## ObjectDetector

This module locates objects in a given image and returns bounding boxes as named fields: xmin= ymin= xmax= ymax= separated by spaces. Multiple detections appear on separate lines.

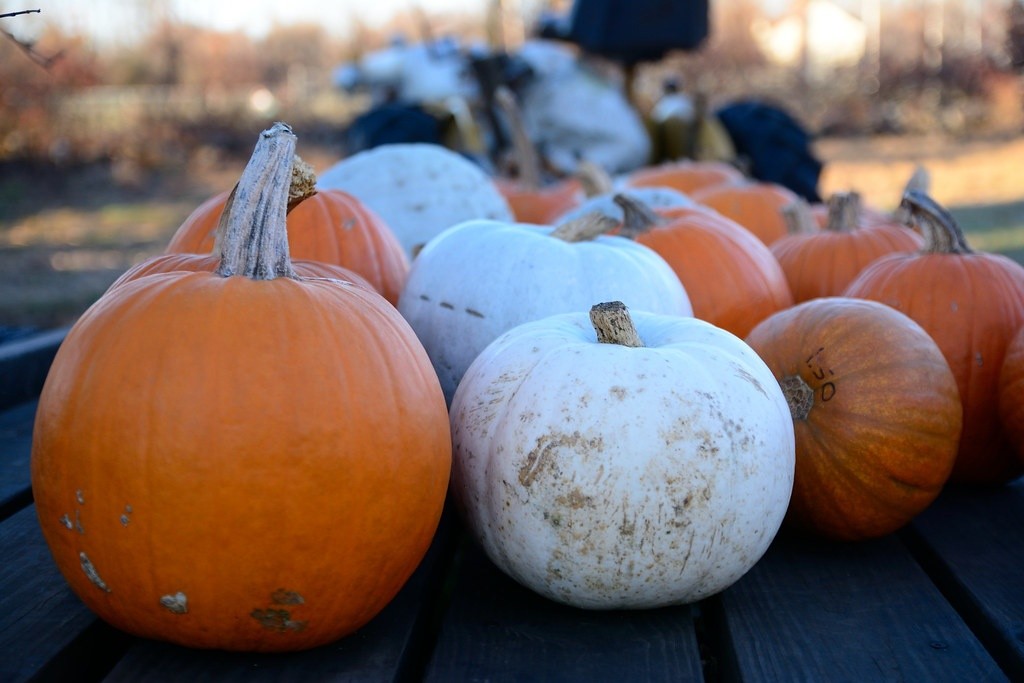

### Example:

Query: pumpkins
xmin=28 ymin=94 xmax=1024 ymax=649
